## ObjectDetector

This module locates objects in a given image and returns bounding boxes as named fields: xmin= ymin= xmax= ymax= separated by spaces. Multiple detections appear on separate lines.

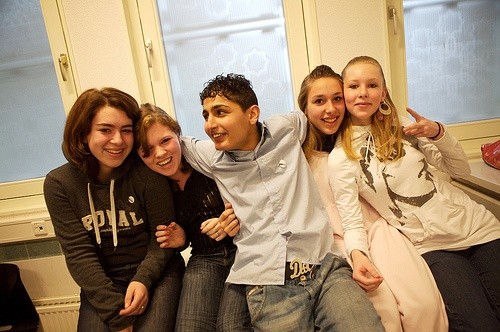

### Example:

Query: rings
xmin=142 ymin=306 xmax=145 ymax=309
xmin=216 ymin=232 xmax=220 ymax=236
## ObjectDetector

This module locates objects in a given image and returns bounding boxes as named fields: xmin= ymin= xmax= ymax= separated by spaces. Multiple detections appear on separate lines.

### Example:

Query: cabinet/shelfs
xmin=450 ymin=158 xmax=500 ymax=221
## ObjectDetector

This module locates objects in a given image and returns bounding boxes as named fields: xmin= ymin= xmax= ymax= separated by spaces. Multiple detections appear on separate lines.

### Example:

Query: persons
xmin=135 ymin=103 xmax=251 ymax=332
xmin=181 ymin=76 xmax=384 ymax=332
xmin=44 ymin=88 xmax=185 ymax=332
xmin=328 ymin=56 xmax=500 ymax=332
xmin=298 ymin=65 xmax=448 ymax=332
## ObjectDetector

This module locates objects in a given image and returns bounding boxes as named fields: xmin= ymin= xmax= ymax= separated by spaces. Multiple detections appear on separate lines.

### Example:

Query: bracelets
xmin=429 ymin=122 xmax=441 ymax=139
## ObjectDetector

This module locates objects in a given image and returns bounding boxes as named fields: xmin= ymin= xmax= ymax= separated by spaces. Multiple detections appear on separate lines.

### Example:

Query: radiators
xmin=31 ymin=294 xmax=81 ymax=332
